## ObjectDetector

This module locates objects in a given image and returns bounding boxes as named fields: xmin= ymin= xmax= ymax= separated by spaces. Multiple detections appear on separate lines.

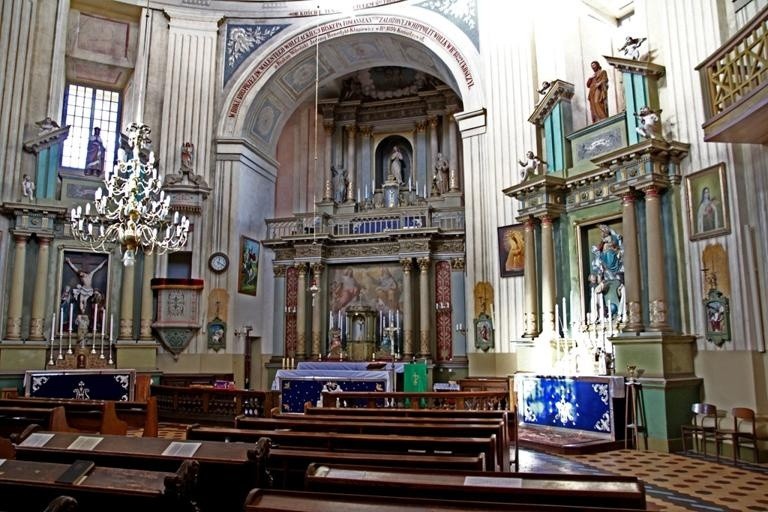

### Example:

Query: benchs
xmin=0 ymin=364 xmax=646 ymax=511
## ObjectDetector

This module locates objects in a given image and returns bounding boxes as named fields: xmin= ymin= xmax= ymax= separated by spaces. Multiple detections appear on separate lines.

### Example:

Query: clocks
xmin=207 ymin=251 xmax=230 ymax=273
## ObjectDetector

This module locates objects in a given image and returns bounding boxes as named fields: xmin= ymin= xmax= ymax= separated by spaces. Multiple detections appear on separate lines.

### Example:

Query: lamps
xmin=65 ymin=0 xmax=193 ymax=267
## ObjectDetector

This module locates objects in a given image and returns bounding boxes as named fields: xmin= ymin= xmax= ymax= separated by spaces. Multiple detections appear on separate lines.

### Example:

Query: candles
xmin=94 ymin=303 xmax=98 ymax=330
xmin=357 ymin=189 xmax=361 ymax=203
xmin=110 ymin=313 xmax=114 ymax=339
xmin=424 ymin=183 xmax=427 ymax=200
xmin=372 ymin=179 xmax=375 ymax=194
xmin=365 ymin=184 xmax=368 ymax=199
xmin=101 ymin=308 xmax=106 ymax=336
xmin=68 ymin=302 xmax=73 ymax=329
xmin=59 ymin=307 xmax=63 ymax=336
xmin=328 ymin=310 xmax=349 ymax=337
xmin=553 ymin=282 xmax=631 ymax=355
xmin=408 ymin=178 xmax=412 ymax=192
xmin=415 ymin=180 xmax=419 ymax=195
xmin=52 ymin=311 xmax=55 ymax=341
xmin=379 ymin=309 xmax=401 ymax=336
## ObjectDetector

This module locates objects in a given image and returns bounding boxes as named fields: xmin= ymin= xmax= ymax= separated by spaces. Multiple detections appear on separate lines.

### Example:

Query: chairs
xmin=680 ymin=399 xmax=720 ymax=462
xmin=714 ymin=403 xmax=760 ymax=465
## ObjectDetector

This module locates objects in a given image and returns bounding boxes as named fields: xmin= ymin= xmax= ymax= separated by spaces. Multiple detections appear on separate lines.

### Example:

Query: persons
xmin=434 ymin=152 xmax=449 ymax=196
xmin=84 ymin=127 xmax=106 ymax=177
xmin=695 ymin=186 xmax=720 ymax=232
xmin=588 ymin=224 xmax=625 ymax=319
xmin=633 ymin=104 xmax=663 ymax=140
xmin=617 ymin=36 xmax=647 ymax=61
xmin=516 ymin=149 xmax=548 ymax=182
xmin=534 ymin=80 xmax=554 ymax=108
xmin=22 ymin=173 xmax=35 ymax=200
xmin=390 ymin=146 xmax=403 ymax=185
xmin=329 ymin=263 xmax=404 ymax=354
xmin=587 ymin=61 xmax=609 ymax=123
xmin=332 ymin=161 xmax=348 ymax=204
xmin=62 ymin=257 xmax=107 ymax=334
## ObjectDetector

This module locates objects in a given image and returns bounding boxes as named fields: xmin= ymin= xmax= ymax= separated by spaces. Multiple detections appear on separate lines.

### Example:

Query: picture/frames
xmin=700 ymin=288 xmax=731 ymax=347
xmin=207 ymin=317 xmax=227 ymax=352
xmin=53 ymin=245 xmax=112 ymax=338
xmin=471 ymin=312 xmax=495 ymax=352
xmin=236 ymin=232 xmax=262 ymax=297
xmin=495 ymin=222 xmax=526 ymax=278
xmin=683 ymin=161 xmax=733 ymax=240
